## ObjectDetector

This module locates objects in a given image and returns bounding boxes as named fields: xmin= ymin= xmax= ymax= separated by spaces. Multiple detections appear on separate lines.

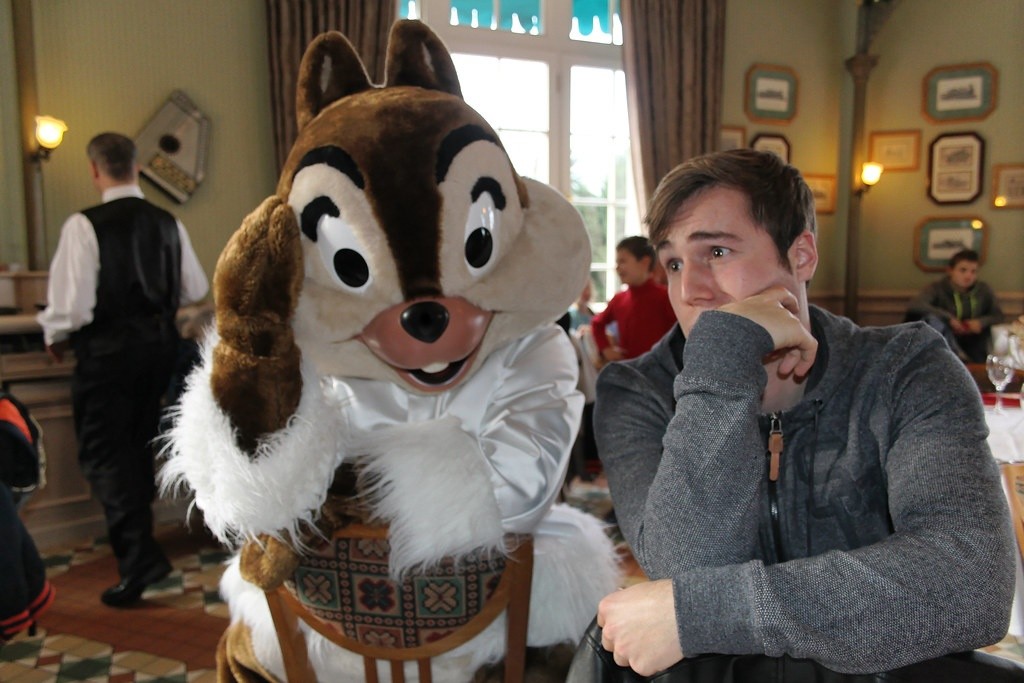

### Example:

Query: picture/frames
xmin=921 ymin=62 xmax=998 ymax=124
xmin=721 ymin=125 xmax=746 ymax=150
xmin=749 ymin=133 xmax=791 ymax=165
xmin=913 ymin=215 xmax=989 ymax=272
xmin=800 ymin=173 xmax=838 ymax=215
xmin=744 ymin=64 xmax=801 ymax=125
xmin=990 ymin=163 xmax=1024 ymax=209
xmin=926 ymin=132 xmax=986 ymax=206
xmin=868 ymin=130 xmax=921 ymax=171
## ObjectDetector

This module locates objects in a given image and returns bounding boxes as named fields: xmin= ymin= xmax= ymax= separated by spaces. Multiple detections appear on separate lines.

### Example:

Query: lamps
xmin=30 ymin=116 xmax=68 ymax=160
xmin=854 ymin=163 xmax=885 ymax=199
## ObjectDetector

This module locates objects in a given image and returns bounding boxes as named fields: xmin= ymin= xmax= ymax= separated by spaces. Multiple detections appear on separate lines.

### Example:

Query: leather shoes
xmin=102 ymin=559 xmax=172 ymax=604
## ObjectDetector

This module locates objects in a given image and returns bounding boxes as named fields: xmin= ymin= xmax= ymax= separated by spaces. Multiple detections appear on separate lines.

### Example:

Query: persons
xmin=35 ymin=132 xmax=209 ymax=607
xmin=909 ymin=250 xmax=1004 ymax=364
xmin=594 ymin=148 xmax=1016 ymax=674
xmin=554 ymin=237 xmax=678 ymax=490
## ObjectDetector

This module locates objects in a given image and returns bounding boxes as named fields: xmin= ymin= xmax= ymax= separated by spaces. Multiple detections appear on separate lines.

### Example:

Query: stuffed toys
xmin=158 ymin=20 xmax=619 ymax=683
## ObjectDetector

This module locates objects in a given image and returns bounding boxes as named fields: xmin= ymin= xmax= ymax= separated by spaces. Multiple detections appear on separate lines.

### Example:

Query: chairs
xmin=265 ymin=524 xmax=533 ymax=683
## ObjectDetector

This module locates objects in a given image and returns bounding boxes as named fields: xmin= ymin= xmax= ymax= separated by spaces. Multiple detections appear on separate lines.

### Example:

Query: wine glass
xmin=985 ymin=354 xmax=1015 ymax=415
